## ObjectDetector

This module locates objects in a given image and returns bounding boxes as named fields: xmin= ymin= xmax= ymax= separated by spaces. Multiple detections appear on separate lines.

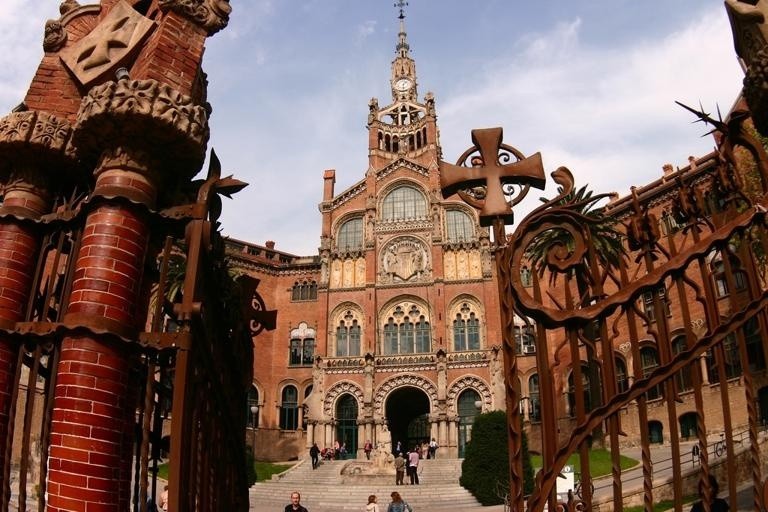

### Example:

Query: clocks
xmin=393 ymin=77 xmax=414 ymax=92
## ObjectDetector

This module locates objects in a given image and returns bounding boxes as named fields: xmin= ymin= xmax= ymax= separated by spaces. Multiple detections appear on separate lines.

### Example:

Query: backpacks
xmin=311 ymin=448 xmax=316 ymax=456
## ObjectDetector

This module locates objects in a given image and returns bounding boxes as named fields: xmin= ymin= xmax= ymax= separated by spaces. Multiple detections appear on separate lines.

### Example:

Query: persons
xmin=404 ymin=450 xmax=411 ymax=485
xmin=688 ymin=472 xmax=730 ymax=511
xmin=396 ymin=451 xmax=405 ymax=484
xmin=408 ymin=445 xmax=419 ymax=485
xmin=386 ymin=491 xmax=412 ymax=512
xmin=365 ymin=494 xmax=380 ymax=512
xmin=284 ymin=492 xmax=308 ymax=511
xmin=310 ymin=441 xmax=321 ymax=469
xmin=320 ymin=438 xmax=348 ymax=460
xmin=364 ymin=438 xmax=373 ymax=461
xmin=394 ymin=436 xmax=438 ymax=459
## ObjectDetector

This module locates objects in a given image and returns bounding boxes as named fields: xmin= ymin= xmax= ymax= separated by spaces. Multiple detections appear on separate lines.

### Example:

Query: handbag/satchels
xmin=404 ymin=505 xmax=409 ymax=512
xmin=435 ymin=444 xmax=439 ymax=449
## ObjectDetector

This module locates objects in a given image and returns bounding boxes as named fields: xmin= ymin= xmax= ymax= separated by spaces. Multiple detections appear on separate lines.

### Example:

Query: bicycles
xmin=574 ymin=472 xmax=595 ymax=500
xmin=715 ymin=431 xmax=740 ymax=458
